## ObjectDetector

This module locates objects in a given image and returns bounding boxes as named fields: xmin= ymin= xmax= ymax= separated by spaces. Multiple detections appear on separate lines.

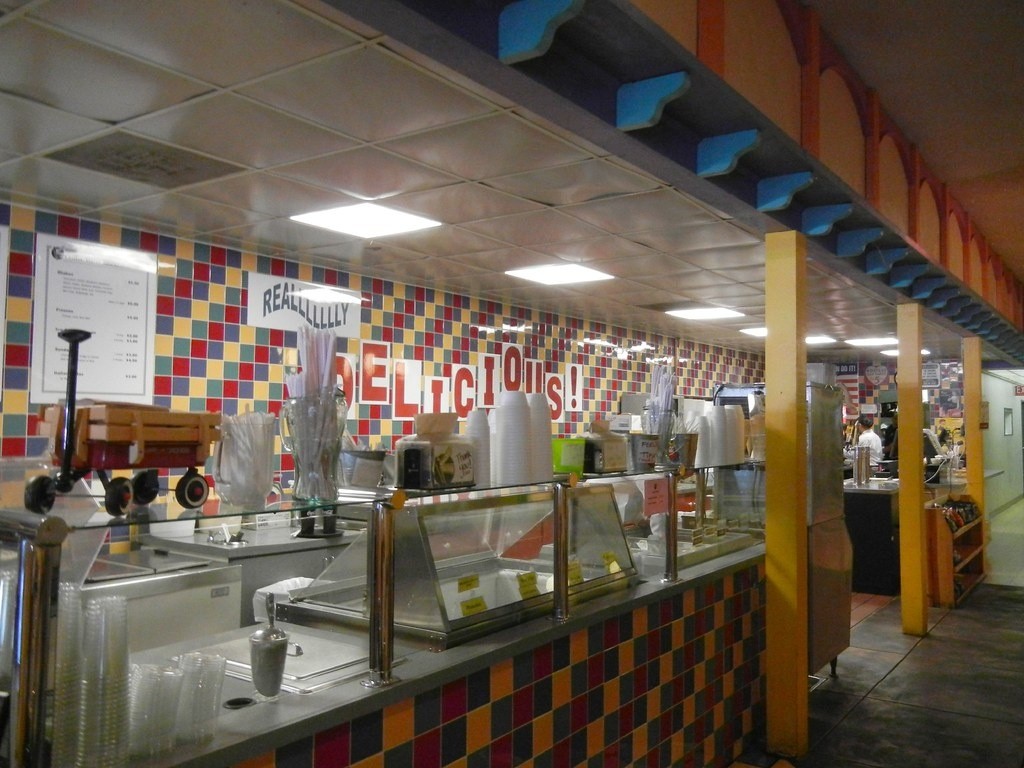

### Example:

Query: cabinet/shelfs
xmin=923 ymin=493 xmax=989 ymax=609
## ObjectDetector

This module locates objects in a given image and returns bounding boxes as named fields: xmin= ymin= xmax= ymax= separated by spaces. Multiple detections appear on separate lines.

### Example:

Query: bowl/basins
xmin=462 ymin=391 xmax=556 ymax=490
xmin=685 ymin=405 xmax=745 ymax=467
xmin=930 ymin=458 xmax=940 ymax=464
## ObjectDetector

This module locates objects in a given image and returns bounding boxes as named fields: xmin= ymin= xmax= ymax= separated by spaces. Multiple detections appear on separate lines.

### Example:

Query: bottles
xmin=852 ymin=445 xmax=870 ymax=485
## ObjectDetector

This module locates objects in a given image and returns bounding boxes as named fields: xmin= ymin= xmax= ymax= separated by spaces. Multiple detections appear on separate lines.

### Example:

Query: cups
xmin=279 ymin=388 xmax=349 ymax=503
xmin=216 ymin=412 xmax=277 ymax=516
xmin=250 ymin=626 xmax=289 ymax=703
xmin=672 ymin=432 xmax=699 ymax=470
xmin=548 ymin=435 xmax=586 ymax=478
xmin=641 ymin=406 xmax=675 ymax=470
xmin=631 ymin=432 xmax=659 ymax=471
xmin=0 ymin=568 xmax=229 ymax=768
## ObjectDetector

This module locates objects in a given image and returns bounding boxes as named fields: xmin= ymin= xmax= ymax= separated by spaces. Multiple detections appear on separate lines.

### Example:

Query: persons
xmin=843 ymin=414 xmax=883 ymax=478
xmin=881 ymin=413 xmax=898 ymax=460
